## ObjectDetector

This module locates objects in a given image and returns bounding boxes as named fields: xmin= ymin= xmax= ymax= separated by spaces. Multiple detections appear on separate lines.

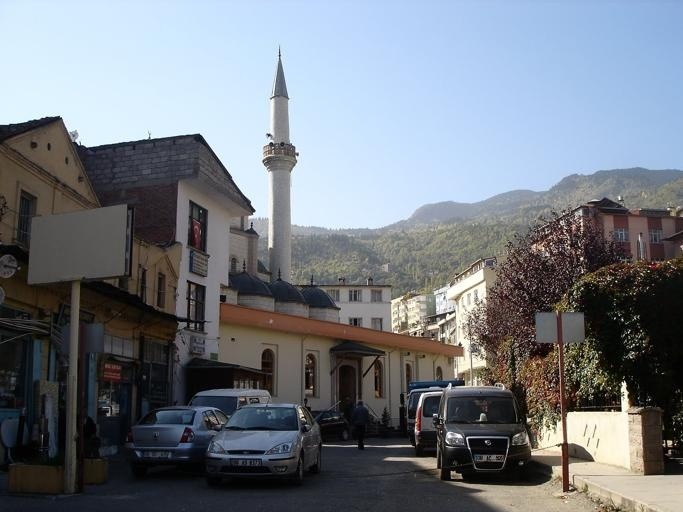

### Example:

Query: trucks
xmin=400 ymin=380 xmax=465 ymax=446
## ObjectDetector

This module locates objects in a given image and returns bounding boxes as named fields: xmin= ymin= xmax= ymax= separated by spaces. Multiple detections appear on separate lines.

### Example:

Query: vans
xmin=415 ymin=392 xmax=444 ymax=457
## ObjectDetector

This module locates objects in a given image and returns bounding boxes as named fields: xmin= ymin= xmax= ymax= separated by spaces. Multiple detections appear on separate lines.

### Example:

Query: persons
xmin=350 ymin=400 xmax=370 ymax=450
xmin=82 ymin=415 xmax=100 ymax=459
xmin=343 ymin=391 xmax=354 ymax=440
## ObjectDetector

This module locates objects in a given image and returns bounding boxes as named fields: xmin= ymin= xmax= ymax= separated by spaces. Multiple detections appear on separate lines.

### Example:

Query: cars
xmin=433 ymin=382 xmax=531 ymax=481
xmin=124 ymin=388 xmax=352 ymax=487
